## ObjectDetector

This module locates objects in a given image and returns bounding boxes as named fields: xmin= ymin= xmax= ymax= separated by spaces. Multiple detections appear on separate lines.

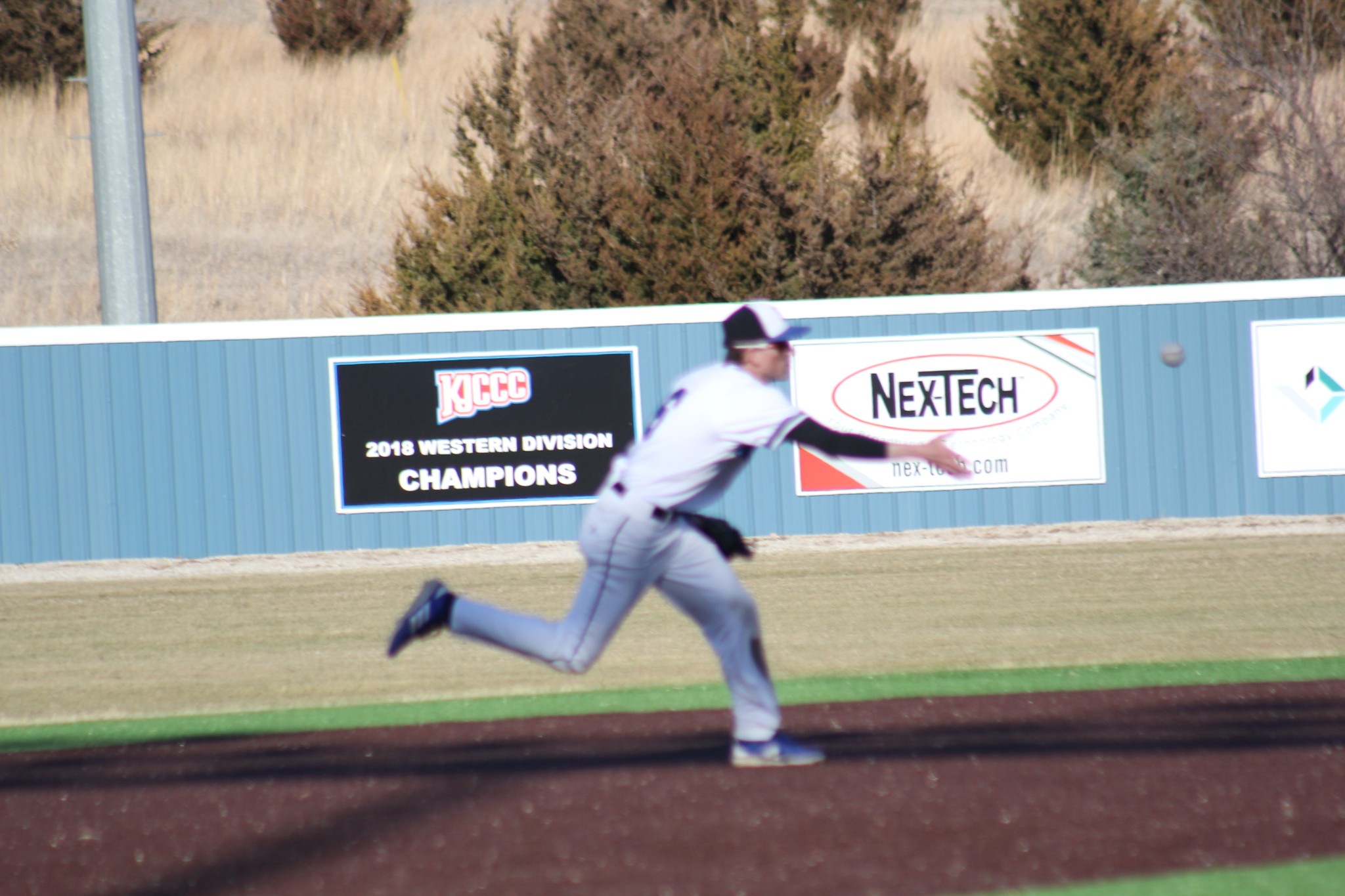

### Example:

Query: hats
xmin=722 ymin=304 xmax=811 ymax=346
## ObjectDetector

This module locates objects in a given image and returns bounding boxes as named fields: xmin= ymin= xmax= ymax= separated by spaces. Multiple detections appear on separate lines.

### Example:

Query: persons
xmin=389 ymin=303 xmax=972 ymax=770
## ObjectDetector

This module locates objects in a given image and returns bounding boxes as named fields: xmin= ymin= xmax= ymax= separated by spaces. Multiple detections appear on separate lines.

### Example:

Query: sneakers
xmin=386 ymin=579 xmax=454 ymax=658
xmin=729 ymin=731 xmax=827 ymax=766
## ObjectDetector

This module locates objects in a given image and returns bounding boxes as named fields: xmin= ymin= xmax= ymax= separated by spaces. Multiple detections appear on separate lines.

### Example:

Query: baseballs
xmin=1160 ymin=342 xmax=1186 ymax=368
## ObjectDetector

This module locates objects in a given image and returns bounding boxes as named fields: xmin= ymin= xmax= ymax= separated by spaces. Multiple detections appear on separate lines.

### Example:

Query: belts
xmin=610 ymin=482 xmax=668 ymax=524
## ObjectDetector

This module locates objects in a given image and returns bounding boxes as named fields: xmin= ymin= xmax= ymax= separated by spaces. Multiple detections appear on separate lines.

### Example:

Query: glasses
xmin=730 ymin=339 xmax=795 ymax=355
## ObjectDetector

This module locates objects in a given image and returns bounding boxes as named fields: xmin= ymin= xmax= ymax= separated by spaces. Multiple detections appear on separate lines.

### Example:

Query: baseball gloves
xmin=693 ymin=513 xmax=754 ymax=560
